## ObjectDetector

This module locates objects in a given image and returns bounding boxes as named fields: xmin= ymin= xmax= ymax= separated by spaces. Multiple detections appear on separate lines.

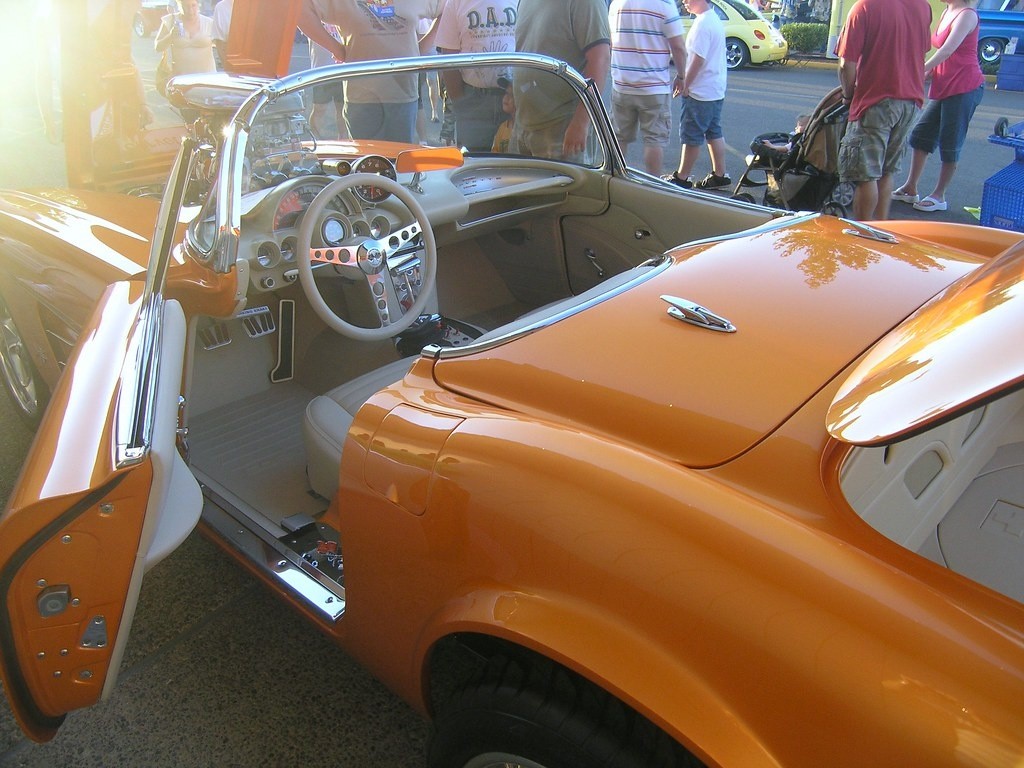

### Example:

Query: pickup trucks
xmin=973 ymin=10 xmax=1023 ymax=77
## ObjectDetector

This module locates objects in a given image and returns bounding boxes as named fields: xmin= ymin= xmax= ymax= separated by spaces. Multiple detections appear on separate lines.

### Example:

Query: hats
xmin=496 ymin=76 xmax=513 ymax=88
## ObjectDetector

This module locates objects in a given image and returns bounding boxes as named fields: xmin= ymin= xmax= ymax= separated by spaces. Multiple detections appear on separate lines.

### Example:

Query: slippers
xmin=913 ymin=195 xmax=948 ymax=213
xmin=892 ymin=187 xmax=920 ymax=204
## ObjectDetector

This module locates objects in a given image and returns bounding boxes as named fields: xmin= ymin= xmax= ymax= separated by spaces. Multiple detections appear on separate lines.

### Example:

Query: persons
xmin=891 ymin=0 xmax=986 ymax=213
xmin=435 ymin=0 xmax=519 ymax=151
xmin=762 ymin=113 xmax=811 ymax=155
xmin=295 ymin=0 xmax=446 ymax=146
xmin=608 ymin=0 xmax=687 ymax=178
xmin=167 ymin=0 xmax=178 ymax=13
xmin=503 ymin=0 xmax=611 ymax=167
xmin=154 ymin=0 xmax=217 ymax=125
xmin=307 ymin=22 xmax=347 ymax=140
xmin=833 ymin=0 xmax=933 ymax=220
xmin=209 ymin=0 xmax=234 ymax=71
xmin=37 ymin=0 xmax=153 ymax=193
xmin=660 ymin=0 xmax=732 ymax=189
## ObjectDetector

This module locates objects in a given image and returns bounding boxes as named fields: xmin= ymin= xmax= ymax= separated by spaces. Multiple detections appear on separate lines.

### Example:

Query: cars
xmin=674 ymin=0 xmax=789 ymax=69
xmin=1 ymin=51 xmax=1024 ymax=767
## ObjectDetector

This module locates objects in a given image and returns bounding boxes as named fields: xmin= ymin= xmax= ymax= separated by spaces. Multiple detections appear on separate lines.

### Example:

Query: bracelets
xmin=677 ymin=75 xmax=684 ymax=80
xmin=841 ymin=93 xmax=853 ymax=100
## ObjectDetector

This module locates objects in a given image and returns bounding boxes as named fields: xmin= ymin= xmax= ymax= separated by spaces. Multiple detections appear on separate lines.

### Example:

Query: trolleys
xmin=980 ymin=117 xmax=1023 ymax=231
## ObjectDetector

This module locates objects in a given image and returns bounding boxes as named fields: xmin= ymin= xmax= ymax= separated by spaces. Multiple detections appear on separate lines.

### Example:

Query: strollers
xmin=726 ymin=85 xmax=855 ymax=224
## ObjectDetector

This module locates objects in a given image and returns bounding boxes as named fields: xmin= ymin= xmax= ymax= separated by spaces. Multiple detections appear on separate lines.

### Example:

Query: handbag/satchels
xmin=155 ymin=49 xmax=174 ymax=97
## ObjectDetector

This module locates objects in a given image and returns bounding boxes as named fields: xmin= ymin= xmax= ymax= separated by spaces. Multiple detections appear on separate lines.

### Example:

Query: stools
xmin=300 ymin=352 xmax=425 ymax=503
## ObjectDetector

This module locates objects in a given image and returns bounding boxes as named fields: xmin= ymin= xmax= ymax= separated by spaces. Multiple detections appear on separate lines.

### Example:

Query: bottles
xmin=1004 ymin=43 xmax=1010 ymax=53
xmin=175 ymin=18 xmax=184 ymax=38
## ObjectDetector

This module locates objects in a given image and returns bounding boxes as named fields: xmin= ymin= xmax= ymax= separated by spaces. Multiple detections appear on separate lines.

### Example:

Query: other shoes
xmin=430 ymin=118 xmax=440 ymax=122
xmin=418 ymin=141 xmax=428 ymax=146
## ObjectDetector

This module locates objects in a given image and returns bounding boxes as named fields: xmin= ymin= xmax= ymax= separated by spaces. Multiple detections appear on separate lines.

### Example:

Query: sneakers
xmin=659 ymin=172 xmax=692 ymax=189
xmin=694 ymin=171 xmax=732 ymax=190
